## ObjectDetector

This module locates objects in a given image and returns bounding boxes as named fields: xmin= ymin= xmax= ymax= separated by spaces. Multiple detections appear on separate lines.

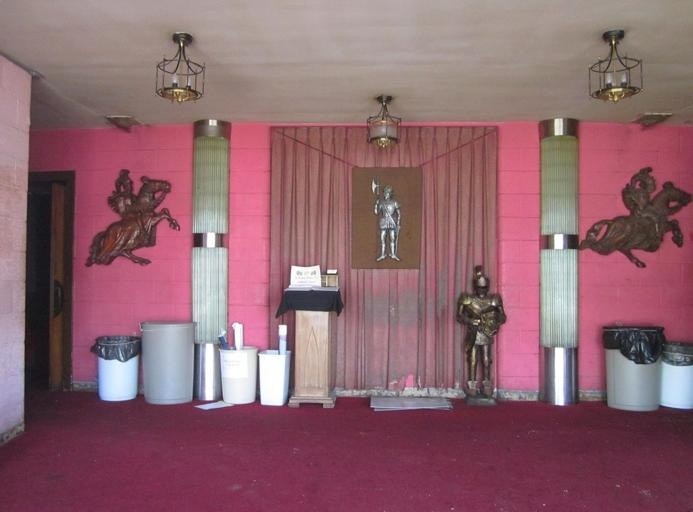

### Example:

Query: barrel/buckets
xmin=139 ymin=320 xmax=198 ymax=407
xmin=216 ymin=346 xmax=260 ymax=406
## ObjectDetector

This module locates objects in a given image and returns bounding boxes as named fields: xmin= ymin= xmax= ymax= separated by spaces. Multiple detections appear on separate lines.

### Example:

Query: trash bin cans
xmin=90 ymin=321 xmax=292 ymax=406
xmin=602 ymin=326 xmax=693 ymax=411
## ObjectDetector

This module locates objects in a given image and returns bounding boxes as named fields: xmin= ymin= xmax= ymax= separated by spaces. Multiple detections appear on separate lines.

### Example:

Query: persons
xmin=106 ymin=168 xmax=152 ymax=241
xmin=457 ymin=265 xmax=507 ymax=397
xmin=621 ymin=166 xmax=666 ymax=238
xmin=373 ymin=184 xmax=402 ymax=262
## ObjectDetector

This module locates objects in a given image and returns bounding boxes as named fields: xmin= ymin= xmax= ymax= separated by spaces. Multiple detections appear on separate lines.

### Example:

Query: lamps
xmin=364 ymin=94 xmax=401 ymax=151
xmin=153 ymin=32 xmax=208 ymax=104
xmin=537 ymin=112 xmax=579 ymax=411
xmin=190 ymin=116 xmax=232 ymax=405
xmin=588 ymin=28 xmax=645 ymax=103
